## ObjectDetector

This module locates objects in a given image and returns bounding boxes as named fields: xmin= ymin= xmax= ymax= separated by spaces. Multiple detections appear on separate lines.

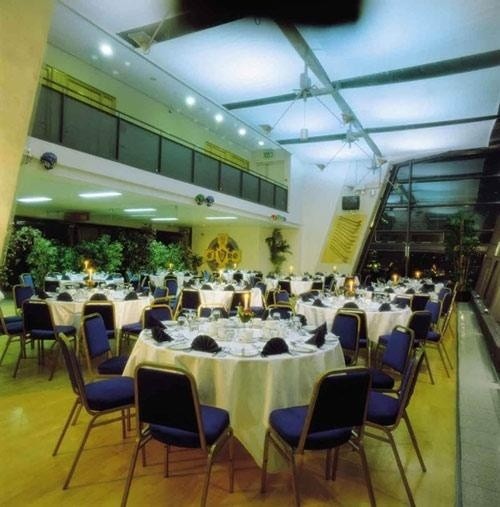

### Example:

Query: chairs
xmin=261 ymin=365 xmax=377 ymax=507
xmin=120 ymin=361 xmax=234 ymax=507
xmin=332 ymin=348 xmax=427 ymax=507
xmin=52 ymin=332 xmax=147 ymax=490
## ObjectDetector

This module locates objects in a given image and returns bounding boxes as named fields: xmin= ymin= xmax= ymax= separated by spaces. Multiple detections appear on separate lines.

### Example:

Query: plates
xmin=229 ymin=346 xmax=259 ymax=357
xmin=292 ymin=344 xmax=317 ymax=353
xmin=324 ymin=335 xmax=338 ymax=342
xmin=303 ymin=326 xmax=318 ymax=332
xmin=166 ymin=343 xmax=191 ymax=350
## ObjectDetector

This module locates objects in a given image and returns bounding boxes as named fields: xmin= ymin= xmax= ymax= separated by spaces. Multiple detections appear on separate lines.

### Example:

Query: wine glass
xmin=266 ymin=307 xmax=273 ymax=321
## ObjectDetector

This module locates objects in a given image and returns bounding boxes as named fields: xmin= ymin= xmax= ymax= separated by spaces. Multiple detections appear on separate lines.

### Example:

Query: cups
xmin=177 ymin=316 xmax=185 ymax=330
xmin=212 ymin=311 xmax=220 ymax=322
xmin=273 ymin=312 xmax=281 ymax=322
